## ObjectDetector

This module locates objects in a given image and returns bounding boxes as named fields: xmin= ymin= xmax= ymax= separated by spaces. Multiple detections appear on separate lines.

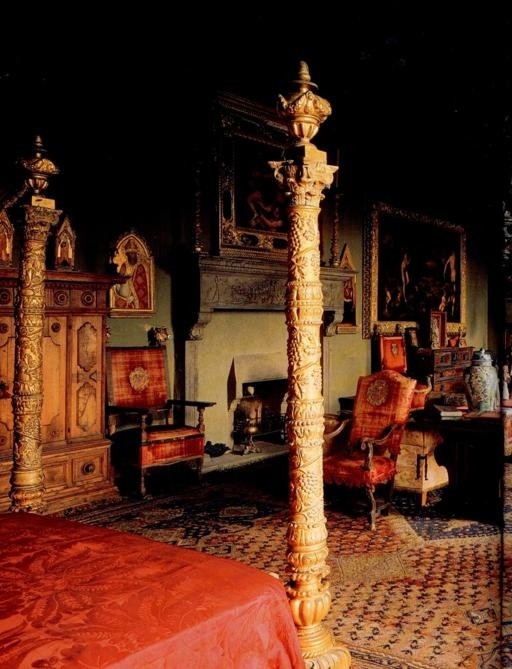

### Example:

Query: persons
xmin=113 ymin=238 xmax=148 ymax=309
xmin=444 ymin=250 xmax=456 ymax=287
xmin=400 ymin=252 xmax=411 ymax=303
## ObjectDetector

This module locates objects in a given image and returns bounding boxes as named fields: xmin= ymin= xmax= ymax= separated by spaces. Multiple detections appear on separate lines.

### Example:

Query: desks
xmin=337 ymin=390 xmax=505 ymax=531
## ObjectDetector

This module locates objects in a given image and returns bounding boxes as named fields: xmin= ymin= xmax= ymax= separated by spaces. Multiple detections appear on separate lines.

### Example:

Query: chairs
xmin=321 ymin=369 xmax=417 ymax=532
xmin=104 ymin=344 xmax=217 ymax=502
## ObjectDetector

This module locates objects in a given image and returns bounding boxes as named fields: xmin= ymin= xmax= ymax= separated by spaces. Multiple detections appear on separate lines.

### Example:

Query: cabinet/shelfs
xmin=412 ymin=346 xmax=476 ymax=393
xmin=0 ymin=267 xmax=128 ymax=516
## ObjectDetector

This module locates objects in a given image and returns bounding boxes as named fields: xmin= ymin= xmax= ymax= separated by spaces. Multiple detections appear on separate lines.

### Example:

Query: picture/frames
xmin=335 ymin=196 xmax=468 ymax=340
xmin=213 ymin=114 xmax=289 ymax=262
xmin=109 ymin=230 xmax=156 ymax=319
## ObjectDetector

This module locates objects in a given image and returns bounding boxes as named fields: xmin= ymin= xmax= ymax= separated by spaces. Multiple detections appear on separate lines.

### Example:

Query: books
xmin=432 ymin=404 xmax=463 ymax=417
